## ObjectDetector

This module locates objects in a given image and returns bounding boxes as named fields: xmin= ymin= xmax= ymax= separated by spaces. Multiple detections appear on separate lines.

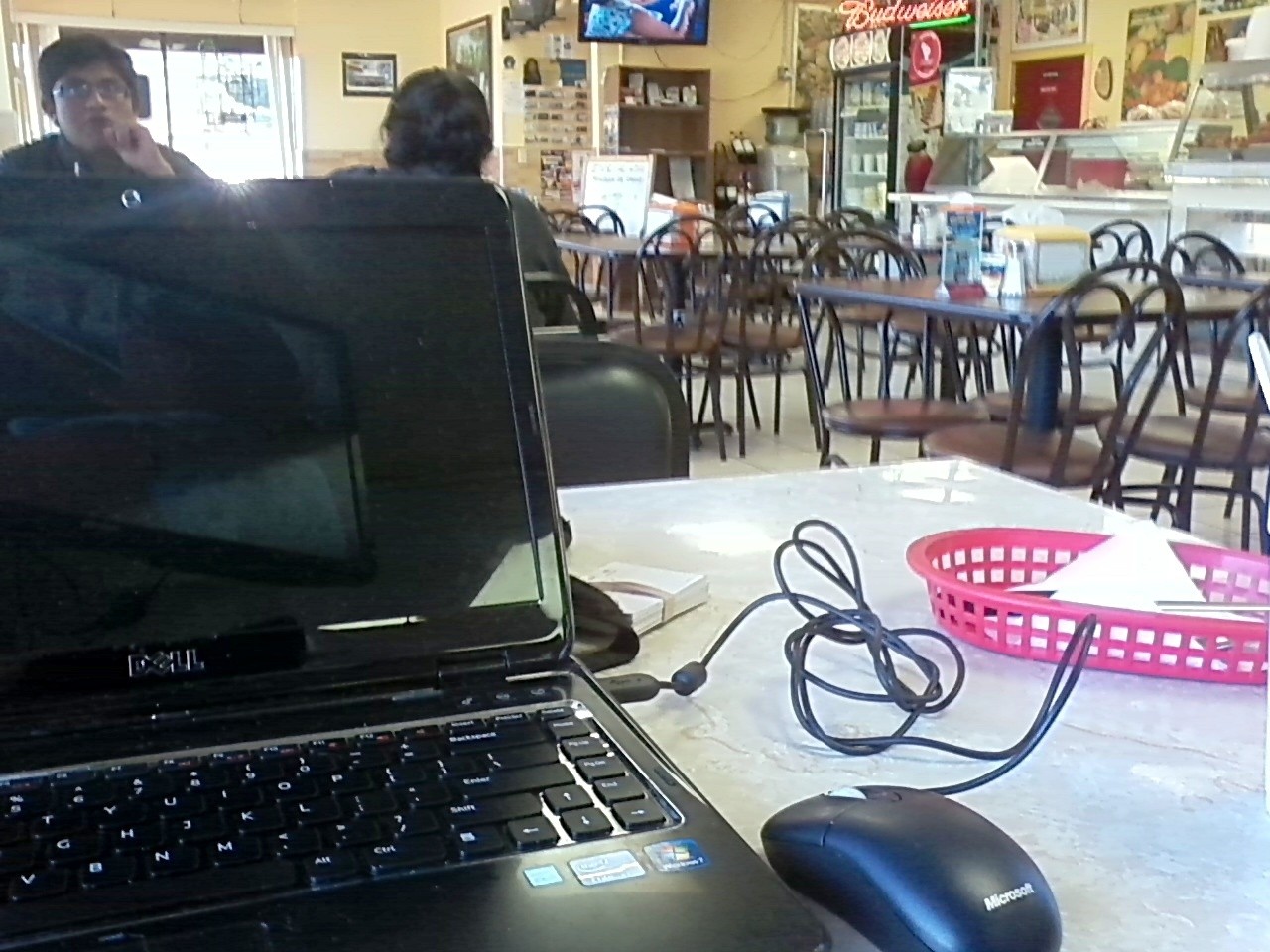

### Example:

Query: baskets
xmin=905 ymin=527 xmax=1269 ymax=687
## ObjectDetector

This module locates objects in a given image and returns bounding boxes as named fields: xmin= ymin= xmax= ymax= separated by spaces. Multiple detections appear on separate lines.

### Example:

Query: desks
xmin=553 ymin=454 xmax=1270 ymax=952
xmin=795 ymin=274 xmax=1268 ymax=429
xmin=558 ymin=231 xmax=810 ymax=440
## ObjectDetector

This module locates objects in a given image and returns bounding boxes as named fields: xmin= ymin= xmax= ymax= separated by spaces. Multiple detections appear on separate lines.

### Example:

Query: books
xmin=580 ymin=560 xmax=710 ymax=636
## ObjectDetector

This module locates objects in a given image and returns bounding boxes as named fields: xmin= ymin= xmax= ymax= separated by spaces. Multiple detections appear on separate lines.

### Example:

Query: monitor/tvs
xmin=577 ymin=0 xmax=710 ymax=45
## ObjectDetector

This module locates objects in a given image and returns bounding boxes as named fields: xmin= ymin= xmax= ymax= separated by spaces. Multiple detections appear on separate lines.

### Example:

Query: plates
xmin=834 ymin=29 xmax=887 ymax=69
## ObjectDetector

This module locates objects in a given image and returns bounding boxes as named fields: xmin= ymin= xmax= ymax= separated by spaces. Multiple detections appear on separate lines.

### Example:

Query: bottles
xmin=912 ymin=204 xmax=939 ymax=247
xmin=903 ymin=139 xmax=934 ymax=231
xmin=764 ymin=114 xmax=800 ymax=145
xmin=716 ymin=129 xmax=757 ymax=210
xmin=1001 ymin=239 xmax=1027 ymax=298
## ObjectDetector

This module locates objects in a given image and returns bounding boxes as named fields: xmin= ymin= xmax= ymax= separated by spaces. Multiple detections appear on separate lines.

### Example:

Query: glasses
xmin=52 ymin=79 xmax=131 ymax=100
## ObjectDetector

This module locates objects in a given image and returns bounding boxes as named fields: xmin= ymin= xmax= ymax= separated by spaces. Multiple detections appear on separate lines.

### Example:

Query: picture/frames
xmin=446 ymin=16 xmax=493 ymax=136
xmin=342 ymin=52 xmax=396 ymax=98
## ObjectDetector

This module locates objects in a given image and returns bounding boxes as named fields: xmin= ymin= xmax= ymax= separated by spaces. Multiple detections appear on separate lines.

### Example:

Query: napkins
xmin=990 ymin=517 xmax=1269 ymax=674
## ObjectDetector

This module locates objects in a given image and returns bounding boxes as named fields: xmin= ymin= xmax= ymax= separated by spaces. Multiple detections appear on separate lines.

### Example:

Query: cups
xmin=981 ymin=259 xmax=1003 ymax=297
xmin=1225 ymin=38 xmax=1245 ymax=62
xmin=843 ymin=80 xmax=890 ymax=216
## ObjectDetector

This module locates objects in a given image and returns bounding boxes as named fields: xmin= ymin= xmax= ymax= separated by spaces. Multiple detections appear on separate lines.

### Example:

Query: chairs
xmin=521 ymin=203 xmax=1270 ymax=562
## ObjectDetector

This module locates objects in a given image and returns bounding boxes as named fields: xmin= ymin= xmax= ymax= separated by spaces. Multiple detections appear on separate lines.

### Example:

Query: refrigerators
xmin=832 ymin=25 xmax=997 ymax=232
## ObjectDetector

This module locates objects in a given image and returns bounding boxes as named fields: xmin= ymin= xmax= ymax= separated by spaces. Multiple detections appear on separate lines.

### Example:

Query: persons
xmin=586 ymin=0 xmax=698 ymax=43
xmin=324 ymin=66 xmax=579 ymax=329
xmin=0 ymin=35 xmax=228 ymax=188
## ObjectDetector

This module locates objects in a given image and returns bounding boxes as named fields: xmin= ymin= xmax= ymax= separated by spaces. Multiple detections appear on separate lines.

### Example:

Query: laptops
xmin=1 ymin=168 xmax=834 ymax=952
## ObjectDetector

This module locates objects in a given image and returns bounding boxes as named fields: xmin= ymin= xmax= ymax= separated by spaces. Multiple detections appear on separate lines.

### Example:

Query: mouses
xmin=760 ymin=780 xmax=1064 ymax=952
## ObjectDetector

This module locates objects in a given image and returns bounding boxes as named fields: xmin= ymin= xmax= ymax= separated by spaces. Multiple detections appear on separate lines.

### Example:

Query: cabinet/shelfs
xmin=605 ymin=65 xmax=712 ymax=205
